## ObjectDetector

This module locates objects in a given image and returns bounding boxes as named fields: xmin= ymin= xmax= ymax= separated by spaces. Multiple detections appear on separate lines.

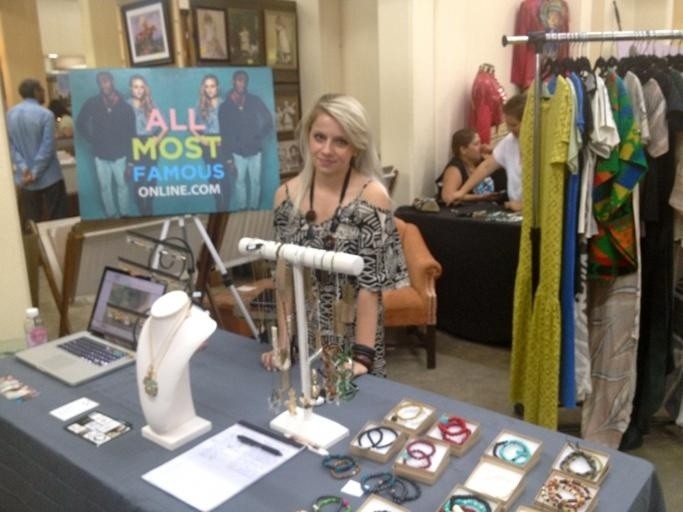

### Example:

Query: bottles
xmin=23 ymin=307 xmax=47 ymax=350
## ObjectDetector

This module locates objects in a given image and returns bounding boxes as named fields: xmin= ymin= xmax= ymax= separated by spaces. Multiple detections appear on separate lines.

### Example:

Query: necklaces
xmin=258 ymin=242 xmax=360 ymax=415
xmin=141 ymin=302 xmax=195 ymax=399
xmin=306 ymin=164 xmax=354 ymax=279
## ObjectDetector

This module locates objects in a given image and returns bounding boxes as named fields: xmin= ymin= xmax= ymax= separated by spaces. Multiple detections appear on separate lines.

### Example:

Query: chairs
xmin=382 ymin=213 xmax=444 ymax=368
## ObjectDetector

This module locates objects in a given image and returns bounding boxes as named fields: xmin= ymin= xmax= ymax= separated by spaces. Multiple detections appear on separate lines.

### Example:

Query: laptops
xmin=13 ymin=265 xmax=169 ymax=387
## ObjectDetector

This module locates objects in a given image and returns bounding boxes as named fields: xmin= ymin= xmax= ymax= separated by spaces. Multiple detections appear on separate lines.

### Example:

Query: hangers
xmin=525 ymin=27 xmax=683 ymax=81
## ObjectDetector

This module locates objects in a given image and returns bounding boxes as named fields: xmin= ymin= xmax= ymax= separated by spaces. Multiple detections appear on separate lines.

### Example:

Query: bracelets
xmin=352 ymin=343 xmax=376 ymax=369
xmin=311 ymin=400 xmax=603 ymax=511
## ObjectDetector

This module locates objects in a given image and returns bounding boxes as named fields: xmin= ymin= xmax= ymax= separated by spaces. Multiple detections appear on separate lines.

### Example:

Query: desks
xmin=0 ymin=325 xmax=666 ymax=512
xmin=393 ymin=205 xmax=520 ymax=348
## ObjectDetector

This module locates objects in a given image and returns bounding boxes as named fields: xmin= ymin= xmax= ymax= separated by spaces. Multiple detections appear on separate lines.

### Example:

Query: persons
xmin=74 ymin=72 xmax=136 ymax=217
xmin=447 ymin=95 xmax=527 ymax=211
xmin=200 ymin=13 xmax=225 ymax=57
xmin=4 ymin=79 xmax=70 ymax=220
xmin=260 ymin=92 xmax=412 ymax=379
xmin=239 ymin=26 xmax=252 ymax=58
xmin=136 ymin=17 xmax=160 ymax=55
xmin=125 ymin=75 xmax=167 ymax=216
xmin=193 ymin=75 xmax=231 ymax=212
xmin=436 ymin=128 xmax=503 ymax=205
xmin=274 ymin=99 xmax=304 ymax=173
xmin=219 ymin=71 xmax=273 ymax=211
xmin=277 ymin=18 xmax=293 ymax=65
xmin=468 ymin=61 xmax=508 ymax=158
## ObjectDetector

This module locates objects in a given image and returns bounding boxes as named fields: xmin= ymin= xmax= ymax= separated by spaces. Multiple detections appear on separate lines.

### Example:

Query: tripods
xmin=147 ymin=215 xmax=266 ymax=345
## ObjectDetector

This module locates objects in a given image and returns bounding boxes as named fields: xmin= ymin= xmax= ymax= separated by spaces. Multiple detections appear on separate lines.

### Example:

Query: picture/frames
xmin=121 ymin=0 xmax=304 ymax=178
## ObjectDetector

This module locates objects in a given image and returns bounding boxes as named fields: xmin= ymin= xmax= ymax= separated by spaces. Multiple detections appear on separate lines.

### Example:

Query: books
xmin=141 ymin=419 xmax=307 ymax=511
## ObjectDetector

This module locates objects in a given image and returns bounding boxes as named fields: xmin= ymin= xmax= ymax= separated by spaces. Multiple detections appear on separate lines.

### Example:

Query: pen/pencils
xmin=237 ymin=435 xmax=282 ymax=456
